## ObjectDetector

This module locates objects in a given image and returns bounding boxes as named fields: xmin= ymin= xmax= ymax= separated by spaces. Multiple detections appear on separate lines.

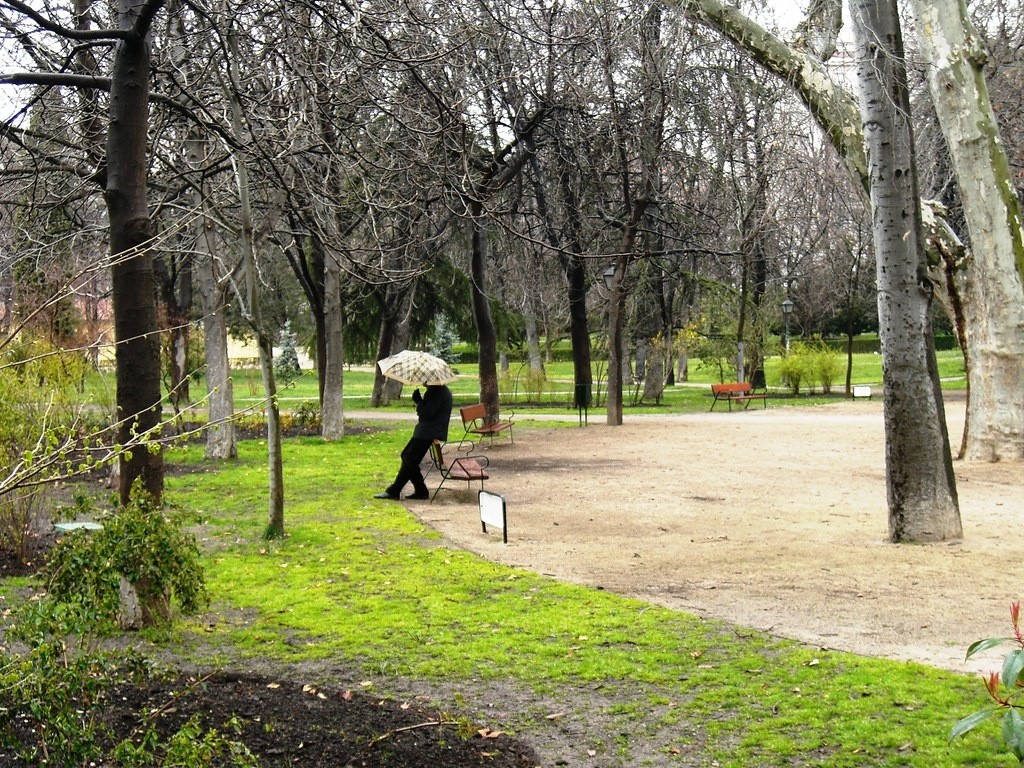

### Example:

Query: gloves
xmin=412 ymin=388 xmax=423 ymax=405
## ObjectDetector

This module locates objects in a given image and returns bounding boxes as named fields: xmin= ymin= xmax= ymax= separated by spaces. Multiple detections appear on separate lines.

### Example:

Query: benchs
xmin=456 ymin=403 xmax=516 ymax=451
xmin=423 ymin=438 xmax=489 ymax=503
xmin=709 ymin=382 xmax=768 ymax=412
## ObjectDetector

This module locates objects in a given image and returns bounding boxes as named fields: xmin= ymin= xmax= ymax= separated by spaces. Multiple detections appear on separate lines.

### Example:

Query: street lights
xmin=780 ymin=298 xmax=794 ymax=388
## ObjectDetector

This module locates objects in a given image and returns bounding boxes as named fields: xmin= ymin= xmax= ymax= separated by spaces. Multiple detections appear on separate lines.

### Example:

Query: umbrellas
xmin=377 ymin=350 xmax=458 ymax=390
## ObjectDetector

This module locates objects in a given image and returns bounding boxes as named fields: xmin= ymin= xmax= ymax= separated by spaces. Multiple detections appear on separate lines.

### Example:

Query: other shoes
xmin=374 ymin=492 xmax=400 ymax=500
xmin=405 ymin=492 xmax=428 ymax=499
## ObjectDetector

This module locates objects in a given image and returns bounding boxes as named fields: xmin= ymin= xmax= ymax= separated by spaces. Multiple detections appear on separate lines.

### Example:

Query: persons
xmin=374 ymin=385 xmax=453 ymax=500
